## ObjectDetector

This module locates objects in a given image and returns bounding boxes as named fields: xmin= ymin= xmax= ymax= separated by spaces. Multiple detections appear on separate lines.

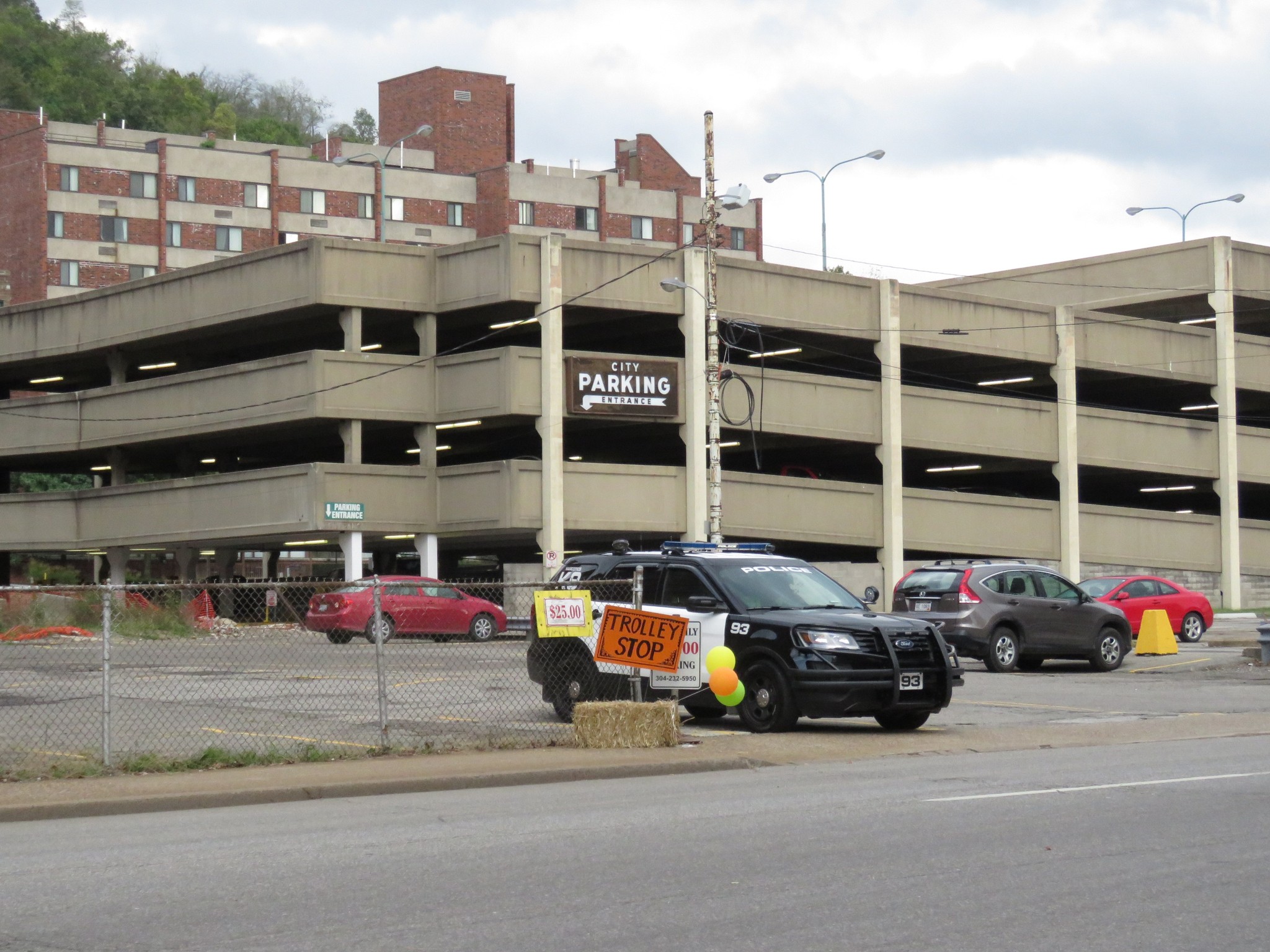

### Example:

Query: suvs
xmin=526 ymin=541 xmax=965 ymax=733
xmin=892 ymin=559 xmax=1133 ymax=674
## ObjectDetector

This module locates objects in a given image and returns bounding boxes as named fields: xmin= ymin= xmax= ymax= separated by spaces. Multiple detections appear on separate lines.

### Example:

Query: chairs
xmin=1010 ymin=577 xmax=1026 ymax=595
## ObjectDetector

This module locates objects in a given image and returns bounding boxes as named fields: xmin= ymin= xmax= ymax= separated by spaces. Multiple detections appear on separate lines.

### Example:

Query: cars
xmin=305 ymin=575 xmax=509 ymax=646
xmin=1052 ymin=575 xmax=1214 ymax=643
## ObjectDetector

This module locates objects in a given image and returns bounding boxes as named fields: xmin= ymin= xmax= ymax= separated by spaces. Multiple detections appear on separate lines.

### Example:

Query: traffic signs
xmin=324 ymin=502 xmax=364 ymax=521
xmin=565 ymin=356 xmax=681 ymax=419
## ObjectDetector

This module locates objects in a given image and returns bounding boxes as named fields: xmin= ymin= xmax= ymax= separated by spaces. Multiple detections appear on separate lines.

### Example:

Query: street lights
xmin=1125 ymin=193 xmax=1245 ymax=242
xmin=659 ymin=279 xmax=723 ymax=552
xmin=763 ymin=149 xmax=886 ymax=272
xmin=332 ymin=124 xmax=433 ymax=245
